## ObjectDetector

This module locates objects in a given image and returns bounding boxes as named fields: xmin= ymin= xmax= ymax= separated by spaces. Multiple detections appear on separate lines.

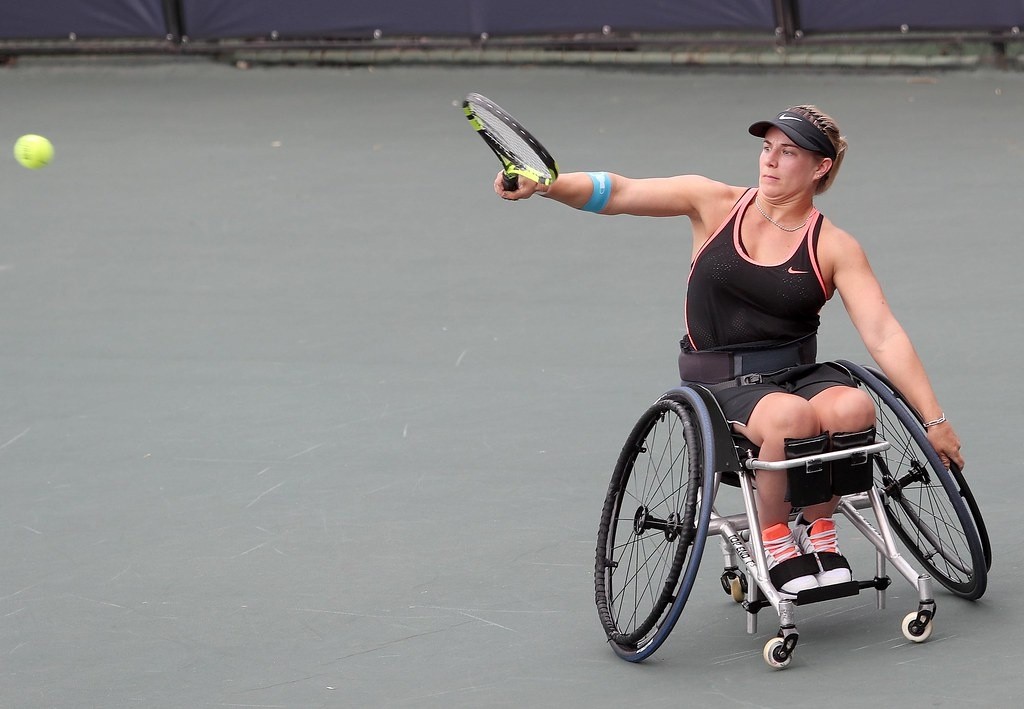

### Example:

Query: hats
xmin=748 ymin=112 xmax=836 ymax=161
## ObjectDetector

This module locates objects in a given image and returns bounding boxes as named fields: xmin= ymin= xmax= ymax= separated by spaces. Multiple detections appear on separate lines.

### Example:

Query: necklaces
xmin=756 ymin=197 xmax=817 ymax=232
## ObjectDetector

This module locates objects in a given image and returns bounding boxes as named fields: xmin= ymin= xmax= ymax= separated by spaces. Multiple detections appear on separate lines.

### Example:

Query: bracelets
xmin=923 ymin=413 xmax=946 ymax=427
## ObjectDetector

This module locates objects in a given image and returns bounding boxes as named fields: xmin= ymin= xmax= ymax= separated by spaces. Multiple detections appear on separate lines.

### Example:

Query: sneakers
xmin=749 ymin=524 xmax=819 ymax=594
xmin=792 ymin=512 xmax=851 ymax=587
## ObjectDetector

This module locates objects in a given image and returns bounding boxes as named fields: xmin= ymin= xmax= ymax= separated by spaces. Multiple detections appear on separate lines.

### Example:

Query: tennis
xmin=14 ymin=133 xmax=56 ymax=169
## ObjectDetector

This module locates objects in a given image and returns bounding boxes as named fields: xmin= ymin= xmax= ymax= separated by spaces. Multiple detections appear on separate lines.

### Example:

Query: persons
xmin=494 ymin=105 xmax=964 ymax=598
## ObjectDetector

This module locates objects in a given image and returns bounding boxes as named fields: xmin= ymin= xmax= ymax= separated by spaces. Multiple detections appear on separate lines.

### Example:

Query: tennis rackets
xmin=463 ymin=92 xmax=560 ymax=202
